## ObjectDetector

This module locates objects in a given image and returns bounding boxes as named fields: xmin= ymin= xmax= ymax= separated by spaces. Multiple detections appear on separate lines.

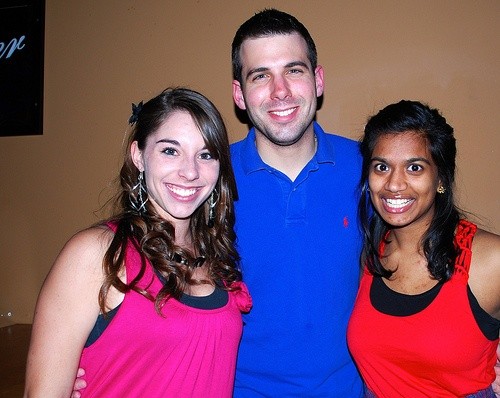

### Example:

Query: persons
xmin=342 ymin=96 xmax=500 ymax=398
xmin=20 ymin=83 xmax=248 ymax=398
xmin=62 ymin=8 xmax=384 ymax=398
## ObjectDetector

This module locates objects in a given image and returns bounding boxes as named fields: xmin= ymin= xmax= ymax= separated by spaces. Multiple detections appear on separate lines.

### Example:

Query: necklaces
xmin=157 ymin=236 xmax=209 ymax=270
